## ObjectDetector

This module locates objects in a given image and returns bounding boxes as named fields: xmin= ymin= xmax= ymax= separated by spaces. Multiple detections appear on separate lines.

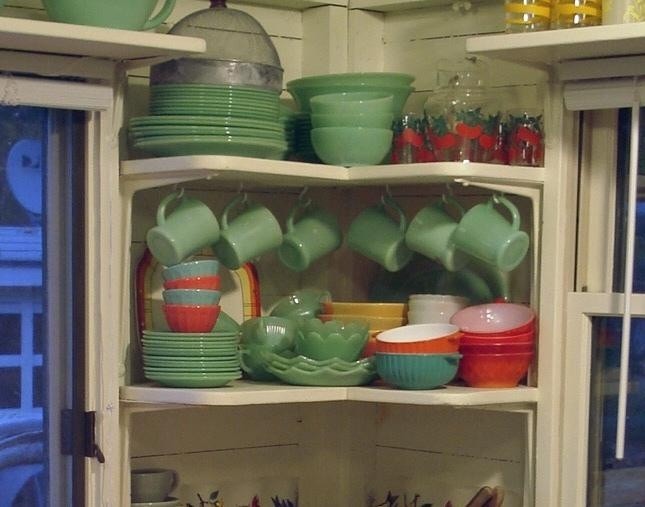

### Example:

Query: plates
xmin=139 ymin=328 xmax=243 ymax=387
xmin=126 ymin=81 xmax=311 ymax=160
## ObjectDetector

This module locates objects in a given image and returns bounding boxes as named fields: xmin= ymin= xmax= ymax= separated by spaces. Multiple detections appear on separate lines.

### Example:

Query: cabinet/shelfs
xmin=117 ymin=156 xmax=546 ymax=408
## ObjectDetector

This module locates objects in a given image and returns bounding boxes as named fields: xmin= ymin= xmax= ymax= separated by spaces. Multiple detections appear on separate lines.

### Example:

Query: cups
xmin=402 ymin=192 xmax=473 ymax=273
xmin=274 ymin=200 xmax=343 ymax=276
xmin=449 ymin=193 xmax=532 ymax=275
xmin=145 ymin=188 xmax=221 ymax=266
xmin=209 ymin=189 xmax=286 ymax=271
xmin=344 ymin=195 xmax=417 ymax=275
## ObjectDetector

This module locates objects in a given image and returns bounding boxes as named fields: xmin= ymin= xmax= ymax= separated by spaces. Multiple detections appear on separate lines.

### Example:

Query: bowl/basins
xmin=159 ymin=260 xmax=224 ymax=332
xmin=131 ymin=466 xmax=178 ymax=502
xmin=240 ymin=285 xmax=537 ymax=389
xmin=285 ymin=70 xmax=419 ymax=115
xmin=306 ymin=86 xmax=397 ymax=166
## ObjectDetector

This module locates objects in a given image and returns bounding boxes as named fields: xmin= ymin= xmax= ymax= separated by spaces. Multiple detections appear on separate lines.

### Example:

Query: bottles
xmin=397 ymin=100 xmax=547 ymax=167
xmin=503 ymin=0 xmax=603 ymax=34
